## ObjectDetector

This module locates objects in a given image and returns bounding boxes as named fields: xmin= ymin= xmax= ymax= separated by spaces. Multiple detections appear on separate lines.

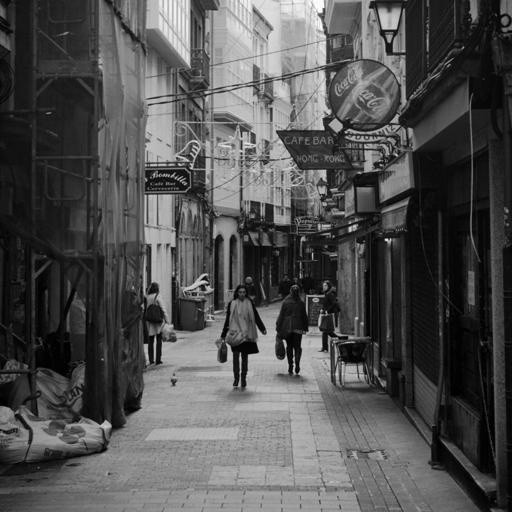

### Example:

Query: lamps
xmin=368 ymin=1 xmax=409 ymax=56
xmin=316 ymin=177 xmax=344 ymax=202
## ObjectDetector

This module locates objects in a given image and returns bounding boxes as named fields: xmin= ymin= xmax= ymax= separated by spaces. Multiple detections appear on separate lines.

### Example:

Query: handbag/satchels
xmin=319 ymin=315 xmax=335 ymax=332
xmin=215 ymin=337 xmax=227 ymax=362
xmin=145 ymin=304 xmax=163 ymax=323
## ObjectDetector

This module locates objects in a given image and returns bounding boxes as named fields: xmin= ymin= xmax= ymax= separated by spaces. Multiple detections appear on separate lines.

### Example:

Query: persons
xmin=244 ymin=273 xmax=307 ymax=305
xmin=320 ymin=280 xmax=344 ymax=352
xmin=218 ymin=284 xmax=266 ymax=386
xmin=273 ymin=286 xmax=308 ymax=375
xmin=139 ymin=282 xmax=168 ymax=366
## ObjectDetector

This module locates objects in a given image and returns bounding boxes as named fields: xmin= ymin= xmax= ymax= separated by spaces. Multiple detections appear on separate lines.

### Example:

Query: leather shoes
xmin=318 ymin=347 xmax=328 ymax=352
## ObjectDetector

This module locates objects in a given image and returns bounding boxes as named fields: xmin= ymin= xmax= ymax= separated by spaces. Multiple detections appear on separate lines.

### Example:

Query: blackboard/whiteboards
xmin=306 ymin=295 xmax=326 ymax=325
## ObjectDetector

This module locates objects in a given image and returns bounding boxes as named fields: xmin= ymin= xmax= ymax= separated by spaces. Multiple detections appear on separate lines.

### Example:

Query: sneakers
xmin=289 ymin=361 xmax=300 ymax=374
xmin=233 ymin=379 xmax=239 ymax=386
xmin=275 ymin=335 xmax=285 ymax=360
xmin=242 ymin=379 xmax=246 ymax=387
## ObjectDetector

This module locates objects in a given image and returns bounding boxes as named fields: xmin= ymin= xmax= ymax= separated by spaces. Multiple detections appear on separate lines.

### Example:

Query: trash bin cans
xmin=179 ymin=297 xmax=205 ymax=330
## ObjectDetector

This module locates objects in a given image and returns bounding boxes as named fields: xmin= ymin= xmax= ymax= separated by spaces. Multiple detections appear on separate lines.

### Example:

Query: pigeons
xmin=171 ymin=373 xmax=178 ymax=386
